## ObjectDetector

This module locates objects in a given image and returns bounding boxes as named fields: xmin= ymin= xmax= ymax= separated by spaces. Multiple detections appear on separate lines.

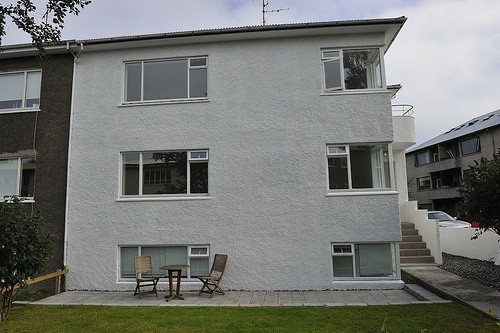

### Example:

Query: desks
xmin=159 ymin=265 xmax=191 ymax=302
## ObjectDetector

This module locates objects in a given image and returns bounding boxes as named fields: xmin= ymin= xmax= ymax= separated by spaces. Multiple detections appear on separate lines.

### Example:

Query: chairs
xmin=199 ymin=254 xmax=228 ymax=299
xmin=133 ymin=256 xmax=159 ymax=301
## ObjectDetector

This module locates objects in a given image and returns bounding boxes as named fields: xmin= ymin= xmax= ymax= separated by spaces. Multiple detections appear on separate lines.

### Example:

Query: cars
xmin=427 ymin=211 xmax=472 ymax=229
